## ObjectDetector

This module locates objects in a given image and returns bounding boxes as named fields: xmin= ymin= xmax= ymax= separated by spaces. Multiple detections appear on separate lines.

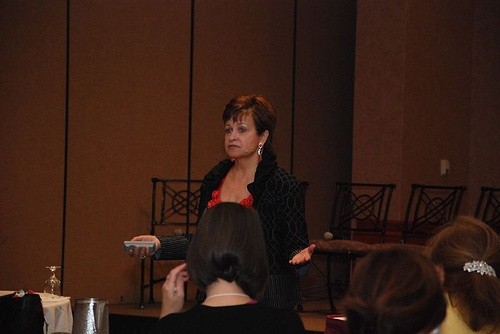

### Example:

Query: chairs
xmin=139 ymin=177 xmax=203 ymax=310
xmin=368 ymin=183 xmax=467 ymax=293
xmin=297 ymin=182 xmax=396 ymax=311
xmin=473 ymin=186 xmax=500 ymax=236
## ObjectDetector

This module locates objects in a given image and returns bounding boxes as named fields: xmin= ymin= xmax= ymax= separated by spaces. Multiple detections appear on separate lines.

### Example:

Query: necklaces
xmin=203 ymin=292 xmax=250 ymax=303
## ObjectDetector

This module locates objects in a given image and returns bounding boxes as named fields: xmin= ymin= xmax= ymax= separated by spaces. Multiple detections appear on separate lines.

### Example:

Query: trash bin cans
xmin=73 ymin=297 xmax=109 ymax=334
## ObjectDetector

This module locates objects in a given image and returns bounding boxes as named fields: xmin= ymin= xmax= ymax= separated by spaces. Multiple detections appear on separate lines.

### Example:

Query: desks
xmin=0 ymin=290 xmax=73 ymax=334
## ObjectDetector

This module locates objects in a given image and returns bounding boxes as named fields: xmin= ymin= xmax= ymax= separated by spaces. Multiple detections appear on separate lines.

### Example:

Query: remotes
xmin=124 ymin=240 xmax=156 ymax=253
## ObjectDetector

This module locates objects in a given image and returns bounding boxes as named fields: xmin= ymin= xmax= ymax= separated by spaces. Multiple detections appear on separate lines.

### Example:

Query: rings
xmin=167 ymin=274 xmax=176 ymax=279
xmin=140 ymin=256 xmax=146 ymax=260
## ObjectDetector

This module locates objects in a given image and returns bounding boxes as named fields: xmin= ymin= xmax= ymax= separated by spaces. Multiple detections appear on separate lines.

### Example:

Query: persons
xmin=128 ymin=95 xmax=316 ymax=311
xmin=159 ymin=201 xmax=306 ymax=334
xmin=346 ymin=245 xmax=448 ymax=334
xmin=418 ymin=215 xmax=500 ymax=334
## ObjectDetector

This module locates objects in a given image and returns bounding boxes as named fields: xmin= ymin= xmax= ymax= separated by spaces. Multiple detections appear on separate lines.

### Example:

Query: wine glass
xmin=44 ymin=266 xmax=61 ymax=300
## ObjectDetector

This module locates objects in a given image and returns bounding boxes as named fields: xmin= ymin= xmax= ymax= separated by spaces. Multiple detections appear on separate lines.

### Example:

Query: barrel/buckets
xmin=72 ymin=298 xmax=109 ymax=334
